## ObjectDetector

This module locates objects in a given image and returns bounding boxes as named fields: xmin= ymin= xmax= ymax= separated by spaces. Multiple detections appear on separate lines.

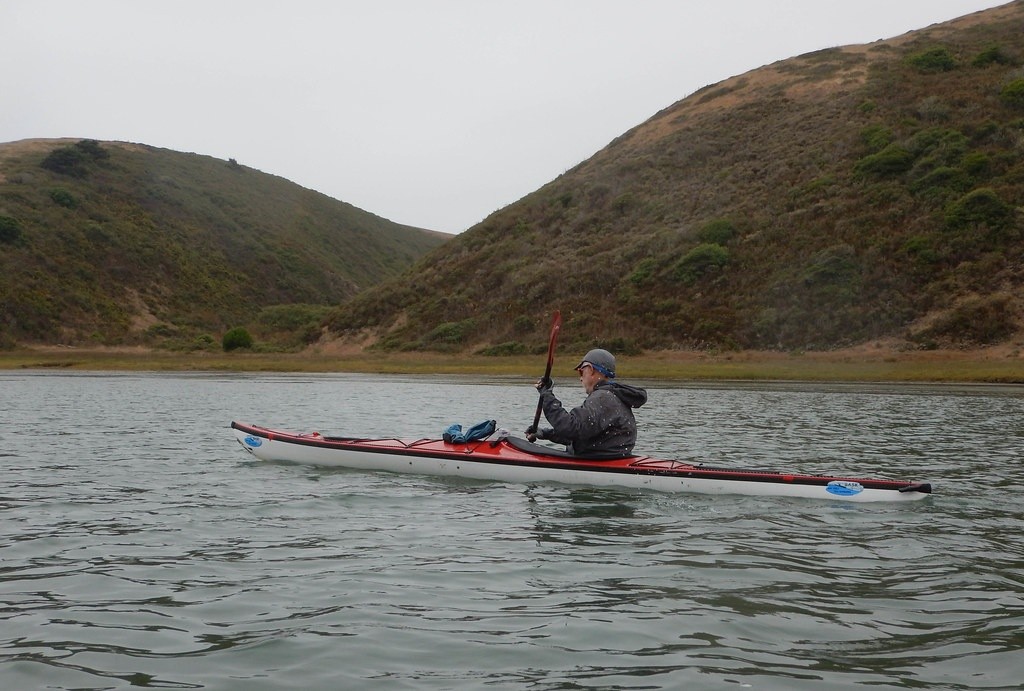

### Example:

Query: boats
xmin=229 ymin=417 xmax=932 ymax=505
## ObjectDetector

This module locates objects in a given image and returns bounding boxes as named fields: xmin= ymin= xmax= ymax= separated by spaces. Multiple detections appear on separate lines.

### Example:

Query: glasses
xmin=577 ymin=362 xmax=592 ymax=376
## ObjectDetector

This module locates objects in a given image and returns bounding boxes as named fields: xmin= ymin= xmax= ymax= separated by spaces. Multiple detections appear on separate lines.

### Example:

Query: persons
xmin=526 ymin=349 xmax=647 ymax=461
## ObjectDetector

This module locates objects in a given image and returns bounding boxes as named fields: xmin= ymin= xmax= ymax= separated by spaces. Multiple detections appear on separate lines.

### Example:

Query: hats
xmin=573 ymin=349 xmax=616 ymax=378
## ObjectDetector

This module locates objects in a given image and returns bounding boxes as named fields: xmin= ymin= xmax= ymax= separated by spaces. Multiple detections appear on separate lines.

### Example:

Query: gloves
xmin=523 ymin=425 xmax=544 ymax=443
xmin=534 ymin=376 xmax=555 ymax=395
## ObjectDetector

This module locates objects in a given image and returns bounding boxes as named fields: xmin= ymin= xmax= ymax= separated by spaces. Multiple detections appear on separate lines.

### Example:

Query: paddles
xmin=528 ymin=310 xmax=561 ymax=444
